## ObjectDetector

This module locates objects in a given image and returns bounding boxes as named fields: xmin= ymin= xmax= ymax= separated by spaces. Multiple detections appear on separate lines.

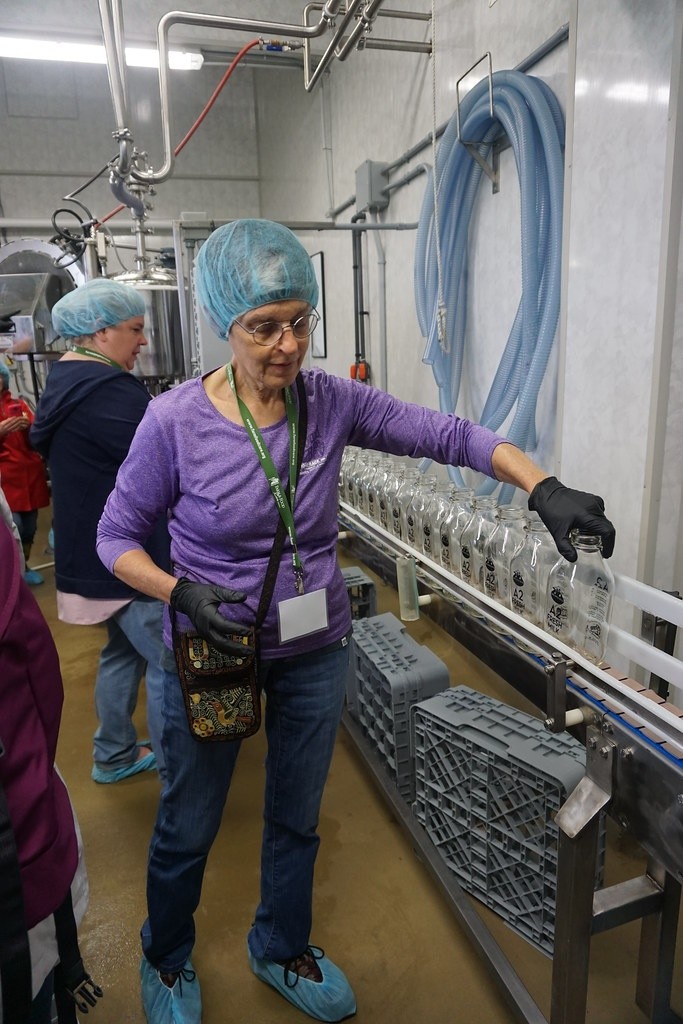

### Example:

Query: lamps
xmin=0 ymin=29 xmax=205 ymax=69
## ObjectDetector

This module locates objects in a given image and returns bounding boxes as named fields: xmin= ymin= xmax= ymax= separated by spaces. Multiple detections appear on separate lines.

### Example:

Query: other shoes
xmin=23 ymin=566 xmax=44 ymax=584
xmin=245 ymin=933 xmax=357 ymax=1021
xmin=91 ymin=740 xmax=157 ymax=784
xmin=138 ymin=953 xmax=202 ymax=1024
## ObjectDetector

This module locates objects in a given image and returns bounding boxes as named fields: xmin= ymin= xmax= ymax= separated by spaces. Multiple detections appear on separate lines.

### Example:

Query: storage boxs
xmin=408 ymin=683 xmax=607 ymax=959
xmin=341 ymin=566 xmax=377 ymax=623
xmin=347 ymin=610 xmax=449 ymax=804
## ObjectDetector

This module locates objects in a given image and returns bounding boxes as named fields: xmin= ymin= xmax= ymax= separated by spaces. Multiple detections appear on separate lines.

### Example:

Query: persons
xmin=0 ymin=357 xmax=50 ymax=584
xmin=96 ymin=219 xmax=615 ymax=1024
xmin=27 ymin=281 xmax=173 ymax=785
xmin=0 ymin=483 xmax=103 ymax=1024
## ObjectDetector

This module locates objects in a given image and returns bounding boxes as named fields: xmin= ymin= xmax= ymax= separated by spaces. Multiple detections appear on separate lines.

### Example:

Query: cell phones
xmin=8 ymin=404 xmax=23 ymax=419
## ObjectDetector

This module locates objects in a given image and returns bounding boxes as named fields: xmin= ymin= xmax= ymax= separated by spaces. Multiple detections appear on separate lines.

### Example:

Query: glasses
xmin=235 ymin=306 xmax=321 ymax=346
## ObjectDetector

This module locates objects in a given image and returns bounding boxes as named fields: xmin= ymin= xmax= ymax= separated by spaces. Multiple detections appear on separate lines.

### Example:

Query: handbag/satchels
xmin=170 ymin=631 xmax=262 ymax=743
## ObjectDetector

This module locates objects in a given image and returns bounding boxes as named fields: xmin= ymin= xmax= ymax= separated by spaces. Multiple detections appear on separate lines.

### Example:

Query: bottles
xmin=337 ymin=445 xmax=615 ymax=669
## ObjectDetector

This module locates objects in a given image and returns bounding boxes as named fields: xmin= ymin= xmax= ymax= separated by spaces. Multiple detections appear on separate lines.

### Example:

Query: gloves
xmin=169 ymin=575 xmax=255 ymax=659
xmin=527 ymin=477 xmax=616 ymax=562
